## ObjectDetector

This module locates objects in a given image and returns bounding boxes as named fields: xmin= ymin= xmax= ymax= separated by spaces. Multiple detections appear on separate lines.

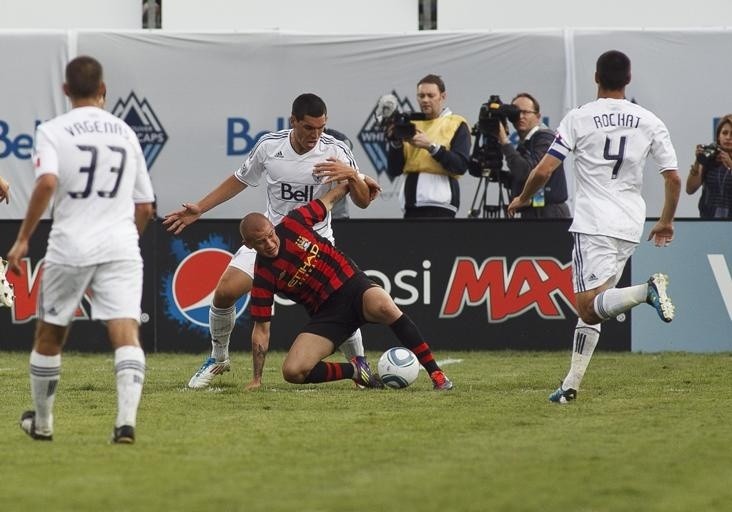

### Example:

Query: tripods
xmin=466 ymin=168 xmax=520 ymax=219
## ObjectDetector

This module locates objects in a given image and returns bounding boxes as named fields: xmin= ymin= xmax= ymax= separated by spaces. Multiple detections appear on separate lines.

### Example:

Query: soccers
xmin=378 ymin=347 xmax=420 ymax=388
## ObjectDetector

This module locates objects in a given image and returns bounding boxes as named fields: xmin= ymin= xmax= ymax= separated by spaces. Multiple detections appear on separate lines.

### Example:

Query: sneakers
xmin=188 ymin=357 xmax=231 ymax=389
xmin=350 ymin=354 xmax=386 ymax=389
xmin=428 ymin=369 xmax=453 ymax=390
xmin=22 ymin=410 xmax=52 ymax=440
xmin=548 ymin=382 xmax=576 ymax=404
xmin=0 ymin=257 xmax=16 ymax=308
xmin=107 ymin=425 xmax=134 ymax=442
xmin=645 ymin=273 xmax=674 ymax=322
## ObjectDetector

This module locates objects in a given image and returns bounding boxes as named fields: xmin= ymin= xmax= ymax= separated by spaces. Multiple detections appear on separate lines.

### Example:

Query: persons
xmin=0 ymin=176 xmax=10 ymax=205
xmin=495 ymin=93 xmax=570 ymax=219
xmin=7 ymin=54 xmax=153 ymax=444
xmin=240 ymin=174 xmax=454 ymax=390
xmin=384 ymin=75 xmax=471 ymax=218
xmin=508 ymin=49 xmax=680 ymax=403
xmin=161 ymin=94 xmax=365 ymax=390
xmin=686 ymin=115 xmax=732 ymax=219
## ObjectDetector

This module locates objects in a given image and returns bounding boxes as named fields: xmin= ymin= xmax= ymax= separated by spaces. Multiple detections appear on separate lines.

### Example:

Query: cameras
xmin=696 ymin=141 xmax=718 ymax=166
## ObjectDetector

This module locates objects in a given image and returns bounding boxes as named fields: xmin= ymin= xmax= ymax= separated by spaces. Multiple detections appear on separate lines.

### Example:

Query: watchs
xmin=428 ymin=141 xmax=438 ymax=154
